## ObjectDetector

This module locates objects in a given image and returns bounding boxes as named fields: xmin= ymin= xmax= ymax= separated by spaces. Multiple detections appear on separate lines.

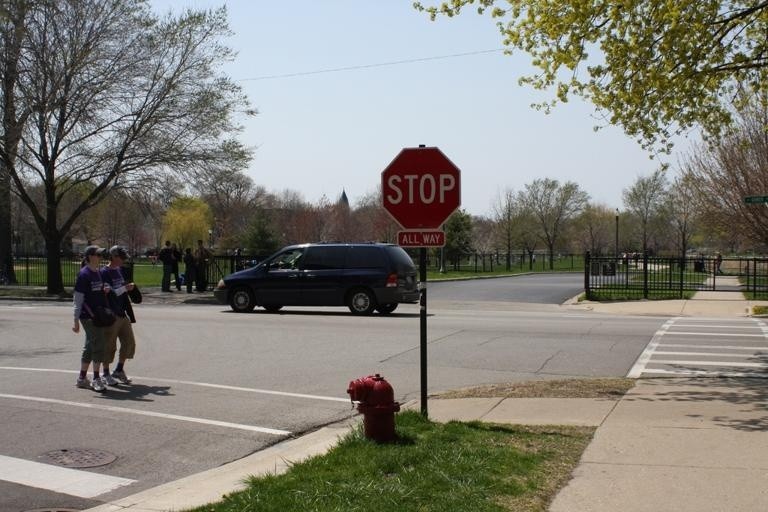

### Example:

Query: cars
xmin=213 ymin=243 xmax=420 ymax=316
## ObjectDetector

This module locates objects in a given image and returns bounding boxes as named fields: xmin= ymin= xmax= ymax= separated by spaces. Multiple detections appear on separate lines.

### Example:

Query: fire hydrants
xmin=348 ymin=374 xmax=399 ymax=442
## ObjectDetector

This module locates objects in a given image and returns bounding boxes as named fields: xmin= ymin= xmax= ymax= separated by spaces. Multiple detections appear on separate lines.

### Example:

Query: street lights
xmin=614 ymin=209 xmax=619 ymax=256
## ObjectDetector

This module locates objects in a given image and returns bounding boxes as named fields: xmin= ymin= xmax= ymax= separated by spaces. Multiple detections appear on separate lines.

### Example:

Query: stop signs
xmin=381 ymin=147 xmax=462 ymax=228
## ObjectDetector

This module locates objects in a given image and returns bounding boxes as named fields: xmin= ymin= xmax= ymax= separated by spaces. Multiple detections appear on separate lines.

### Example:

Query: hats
xmin=109 ymin=244 xmax=131 ymax=260
xmin=84 ymin=245 xmax=106 ymax=255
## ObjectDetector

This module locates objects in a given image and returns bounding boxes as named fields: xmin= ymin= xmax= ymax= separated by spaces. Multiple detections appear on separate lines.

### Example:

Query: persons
xmin=70 ymin=246 xmax=112 ymax=392
xmin=151 ymin=240 xmax=215 ymax=294
xmin=102 ymin=245 xmax=142 ymax=388
xmin=529 ymin=250 xmax=726 ymax=276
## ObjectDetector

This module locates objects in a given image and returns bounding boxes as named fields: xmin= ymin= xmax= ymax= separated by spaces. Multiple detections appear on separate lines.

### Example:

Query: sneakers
xmin=91 ymin=377 xmax=107 ymax=392
xmin=77 ymin=376 xmax=94 ymax=389
xmin=111 ymin=370 xmax=132 ymax=384
xmin=102 ymin=373 xmax=118 ymax=386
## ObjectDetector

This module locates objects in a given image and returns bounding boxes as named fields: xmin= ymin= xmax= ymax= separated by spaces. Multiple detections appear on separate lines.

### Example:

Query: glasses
xmin=91 ymin=254 xmax=101 ymax=257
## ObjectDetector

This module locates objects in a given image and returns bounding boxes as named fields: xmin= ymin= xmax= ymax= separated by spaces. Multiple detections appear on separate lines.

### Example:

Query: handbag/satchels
xmin=90 ymin=306 xmax=117 ymax=327
